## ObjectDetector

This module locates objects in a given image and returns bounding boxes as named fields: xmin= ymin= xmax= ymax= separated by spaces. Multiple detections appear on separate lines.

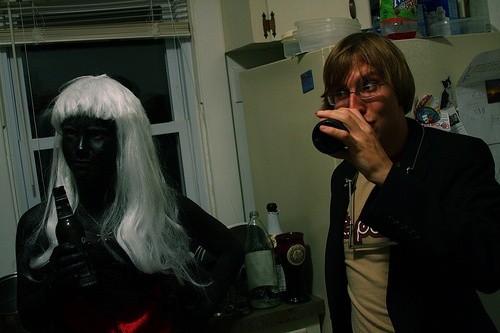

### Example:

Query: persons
xmin=12 ymin=74 xmax=241 ymax=333
xmin=313 ymin=29 xmax=500 ymax=333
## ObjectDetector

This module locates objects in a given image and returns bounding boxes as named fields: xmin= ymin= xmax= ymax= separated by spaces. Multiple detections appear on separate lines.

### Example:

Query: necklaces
xmin=75 ymin=200 xmax=123 ymax=245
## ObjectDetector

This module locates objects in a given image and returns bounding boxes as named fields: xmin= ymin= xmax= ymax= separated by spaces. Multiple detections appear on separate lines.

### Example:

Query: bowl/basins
xmin=429 ymin=15 xmax=490 ymax=37
xmin=224 ymin=39 xmax=285 ymax=69
xmin=281 ymin=16 xmax=361 ymax=58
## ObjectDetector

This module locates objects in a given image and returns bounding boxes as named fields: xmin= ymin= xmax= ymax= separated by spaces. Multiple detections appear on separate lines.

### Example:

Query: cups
xmin=312 ymin=118 xmax=349 ymax=155
xmin=274 ymin=232 xmax=312 ymax=303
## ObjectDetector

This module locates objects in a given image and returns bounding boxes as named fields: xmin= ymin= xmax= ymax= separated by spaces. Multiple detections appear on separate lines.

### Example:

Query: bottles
xmin=379 ymin=0 xmax=417 ymax=39
xmin=52 ymin=186 xmax=99 ymax=287
xmin=244 ymin=211 xmax=280 ymax=309
xmin=265 ymin=202 xmax=286 ymax=302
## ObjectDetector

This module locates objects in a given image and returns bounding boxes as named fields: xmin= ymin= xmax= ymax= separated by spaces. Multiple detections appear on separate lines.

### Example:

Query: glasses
xmin=321 ymin=80 xmax=389 ymax=105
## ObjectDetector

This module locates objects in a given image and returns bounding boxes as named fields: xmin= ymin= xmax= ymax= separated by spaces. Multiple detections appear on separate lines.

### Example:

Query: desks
xmin=241 ymin=292 xmax=325 ymax=333
